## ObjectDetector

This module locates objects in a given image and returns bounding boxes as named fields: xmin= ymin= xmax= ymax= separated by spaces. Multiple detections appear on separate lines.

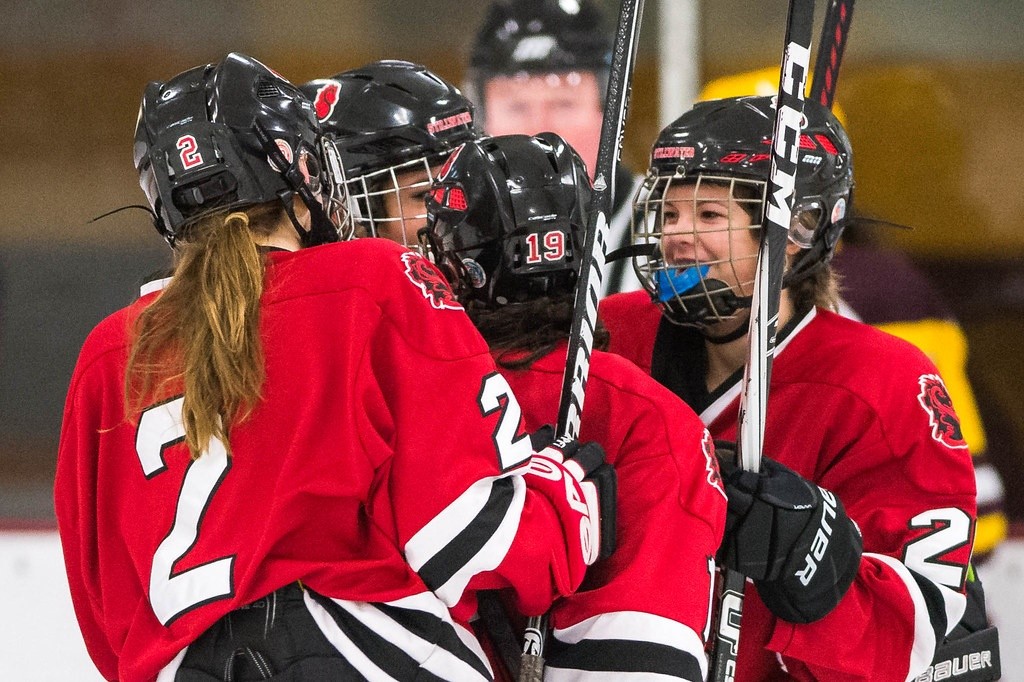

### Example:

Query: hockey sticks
xmin=710 ymin=0 xmax=816 ymax=682
xmin=516 ymin=0 xmax=646 ymax=682
xmin=807 ymin=0 xmax=854 ymax=112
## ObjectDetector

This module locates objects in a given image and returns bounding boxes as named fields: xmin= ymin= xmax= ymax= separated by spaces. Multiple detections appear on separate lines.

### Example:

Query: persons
xmin=698 ymin=66 xmax=1007 ymax=564
xmin=464 ymin=0 xmax=647 ymax=215
xmin=57 ymin=53 xmax=1001 ymax=682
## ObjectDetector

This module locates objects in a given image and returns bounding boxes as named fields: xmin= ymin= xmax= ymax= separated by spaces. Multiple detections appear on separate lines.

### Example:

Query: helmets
xmin=467 ymin=0 xmax=610 ymax=131
xmin=132 ymin=51 xmax=355 ymax=246
xmin=628 ymin=94 xmax=856 ymax=327
xmin=293 ymin=58 xmax=474 ymax=254
xmin=420 ymin=131 xmax=594 ymax=315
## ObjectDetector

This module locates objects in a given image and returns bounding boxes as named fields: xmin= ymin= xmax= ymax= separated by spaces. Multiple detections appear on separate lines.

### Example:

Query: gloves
xmin=715 ymin=441 xmax=865 ymax=624
xmin=528 ymin=424 xmax=616 ymax=565
